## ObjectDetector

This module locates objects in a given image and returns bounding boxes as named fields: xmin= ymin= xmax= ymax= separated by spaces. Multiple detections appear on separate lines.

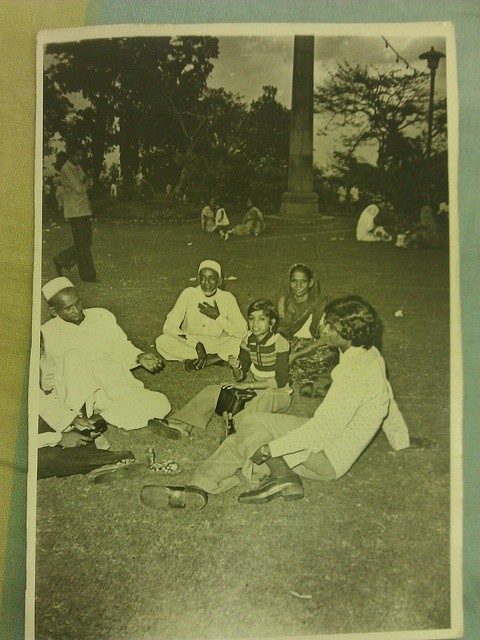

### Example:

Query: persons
xmin=36 ymin=260 xmax=436 ymax=514
xmin=337 ymin=182 xmax=447 ymax=249
xmin=54 ymin=141 xmax=102 ymax=283
xmin=200 ymin=197 xmax=267 ymax=241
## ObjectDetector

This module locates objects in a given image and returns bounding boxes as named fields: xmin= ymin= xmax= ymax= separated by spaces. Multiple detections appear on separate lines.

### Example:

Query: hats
xmin=41 ymin=277 xmax=74 ymax=302
xmin=197 ymin=260 xmax=222 ymax=280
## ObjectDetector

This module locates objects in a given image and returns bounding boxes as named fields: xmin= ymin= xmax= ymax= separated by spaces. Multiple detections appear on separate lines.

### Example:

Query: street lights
xmin=418 ymin=45 xmax=446 ymax=228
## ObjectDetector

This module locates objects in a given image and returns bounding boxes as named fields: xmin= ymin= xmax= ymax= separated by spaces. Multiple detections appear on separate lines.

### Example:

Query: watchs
xmin=260 ymin=446 xmax=269 ymax=461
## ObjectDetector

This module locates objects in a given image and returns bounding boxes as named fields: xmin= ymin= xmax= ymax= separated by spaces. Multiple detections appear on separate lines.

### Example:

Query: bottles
xmin=220 ymin=411 xmax=227 ymax=445
xmin=61 ymin=419 xmax=108 ymax=450
xmin=145 ymin=448 xmax=154 ymax=468
xmin=227 ymin=413 xmax=236 ymax=437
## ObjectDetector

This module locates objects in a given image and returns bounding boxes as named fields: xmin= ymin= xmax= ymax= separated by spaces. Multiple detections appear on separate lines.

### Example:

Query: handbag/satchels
xmin=214 ymin=385 xmax=257 ymax=416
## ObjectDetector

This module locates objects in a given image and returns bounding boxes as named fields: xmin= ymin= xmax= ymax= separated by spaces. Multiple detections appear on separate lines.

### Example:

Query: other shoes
xmin=224 ymin=233 xmax=229 ymax=240
xmin=140 ymin=483 xmax=207 ymax=512
xmin=219 ymin=233 xmax=225 ymax=240
xmin=53 ymin=254 xmax=63 ymax=276
xmin=184 ymin=359 xmax=196 ymax=372
xmin=85 ymin=277 xmax=101 ymax=283
xmin=298 ymin=382 xmax=314 ymax=396
xmin=87 ymin=460 xmax=146 ymax=484
xmin=238 ymin=474 xmax=304 ymax=504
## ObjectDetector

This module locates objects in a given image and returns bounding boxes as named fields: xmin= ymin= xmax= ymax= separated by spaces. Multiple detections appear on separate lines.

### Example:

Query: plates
xmin=148 ymin=466 xmax=181 ymax=474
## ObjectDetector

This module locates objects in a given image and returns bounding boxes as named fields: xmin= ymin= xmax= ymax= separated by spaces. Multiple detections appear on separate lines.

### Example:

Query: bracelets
xmin=250 ymin=381 xmax=254 ymax=390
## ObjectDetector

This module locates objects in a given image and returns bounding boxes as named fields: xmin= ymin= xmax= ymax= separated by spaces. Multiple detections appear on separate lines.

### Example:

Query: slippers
xmin=148 ymin=418 xmax=182 ymax=439
xmin=219 ymin=411 xmax=236 ymax=445
